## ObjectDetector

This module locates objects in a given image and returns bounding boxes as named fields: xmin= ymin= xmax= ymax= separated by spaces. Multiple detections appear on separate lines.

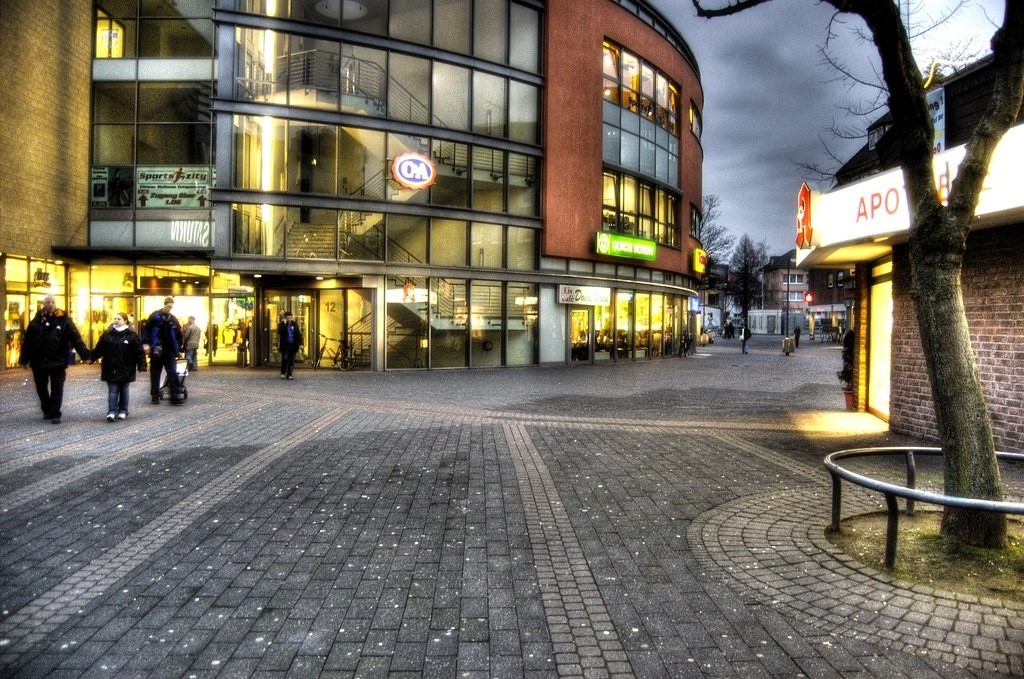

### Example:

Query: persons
xmin=836 ymin=322 xmax=845 ymax=345
xmin=739 ymin=325 xmax=751 ymax=354
xmin=109 ymin=168 xmax=128 ymax=205
xmin=729 ymin=323 xmax=734 ymax=338
xmin=794 ymin=324 xmax=801 ymax=348
xmin=182 ymin=316 xmax=201 ymax=370
xmin=277 ymin=312 xmax=304 ymax=380
xmin=18 ymin=296 xmax=91 ymax=424
xmin=142 ymin=297 xmax=184 ymax=404
xmin=724 ymin=324 xmax=729 ymax=339
xmin=90 ymin=313 xmax=147 ymax=421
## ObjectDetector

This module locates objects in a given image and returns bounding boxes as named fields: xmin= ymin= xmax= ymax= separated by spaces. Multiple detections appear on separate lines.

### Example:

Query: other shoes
xmin=188 ymin=363 xmax=193 ymax=371
xmin=171 ymin=398 xmax=185 ymax=404
xmin=281 ymin=374 xmax=286 ymax=378
xmin=151 ymin=396 xmax=159 ymax=404
xmin=118 ymin=412 xmax=126 ymax=419
xmin=44 ymin=413 xmax=52 ymax=419
xmin=52 ymin=417 xmax=60 ymax=424
xmin=106 ymin=413 xmax=116 ymax=422
xmin=289 ymin=376 xmax=293 ymax=380
xmin=194 ymin=368 xmax=197 ymax=371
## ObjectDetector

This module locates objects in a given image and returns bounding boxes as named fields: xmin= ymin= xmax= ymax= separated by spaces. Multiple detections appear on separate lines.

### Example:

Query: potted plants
xmin=836 ymin=329 xmax=855 ymax=410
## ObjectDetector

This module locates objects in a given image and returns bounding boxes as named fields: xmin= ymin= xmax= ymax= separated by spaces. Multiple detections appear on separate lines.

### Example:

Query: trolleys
xmin=159 ymin=356 xmax=192 ymax=401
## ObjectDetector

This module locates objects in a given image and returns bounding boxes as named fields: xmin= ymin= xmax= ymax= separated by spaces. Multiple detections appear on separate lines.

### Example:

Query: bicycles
xmin=315 ymin=333 xmax=357 ymax=371
xmin=677 ymin=333 xmax=694 ymax=358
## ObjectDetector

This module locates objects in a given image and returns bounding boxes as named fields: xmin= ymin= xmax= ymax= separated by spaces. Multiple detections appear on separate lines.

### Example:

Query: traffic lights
xmin=803 ymin=290 xmax=814 ymax=303
